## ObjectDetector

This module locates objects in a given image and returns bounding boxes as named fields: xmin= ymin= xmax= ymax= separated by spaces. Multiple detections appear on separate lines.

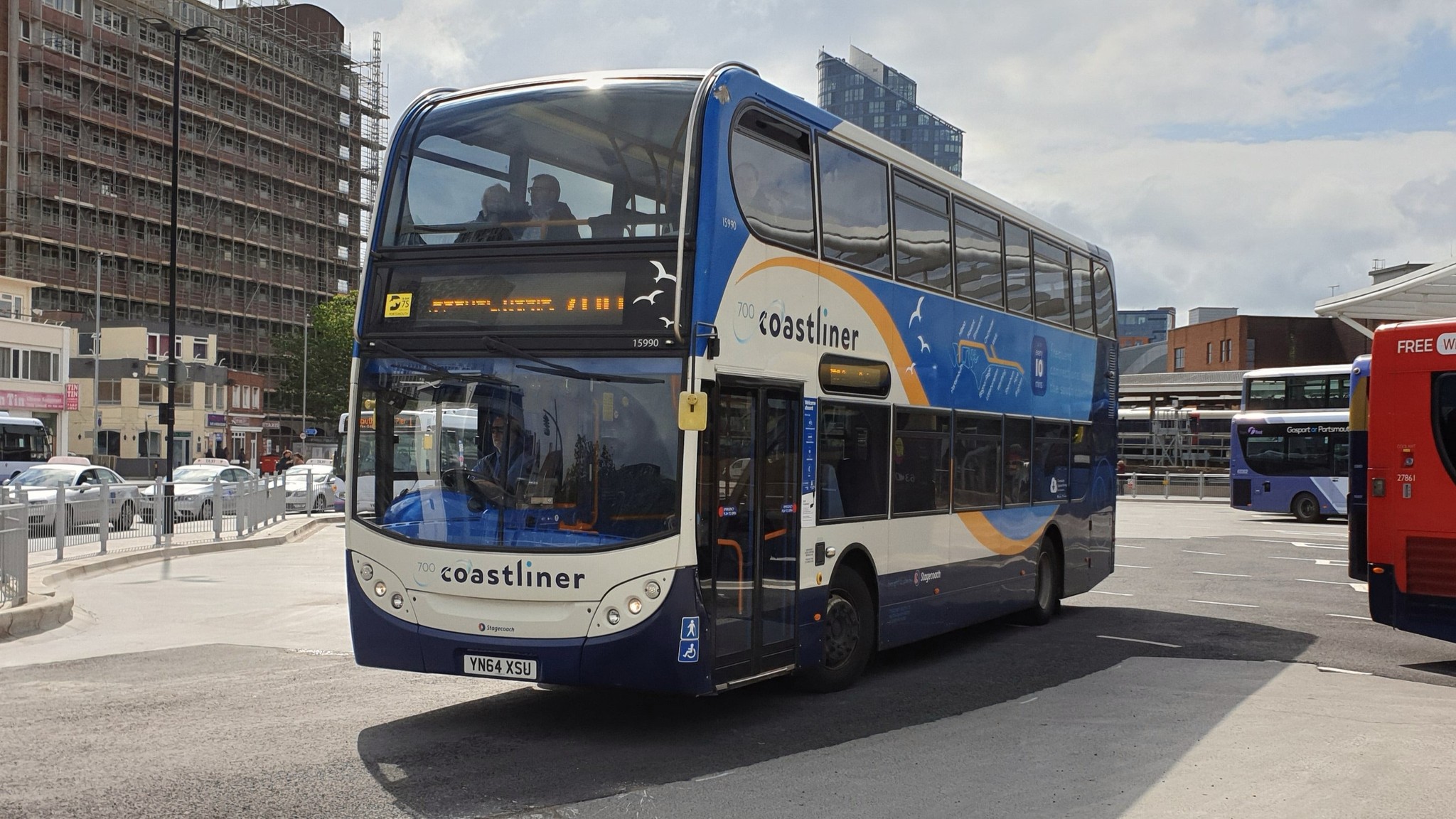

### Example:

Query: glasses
xmin=528 ymin=186 xmax=553 ymax=194
xmin=491 ymin=426 xmax=506 ymax=434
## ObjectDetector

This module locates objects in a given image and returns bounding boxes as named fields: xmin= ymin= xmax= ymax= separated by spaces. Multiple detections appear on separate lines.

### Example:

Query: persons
xmin=276 ymin=450 xmax=305 ymax=475
xmin=453 ymin=174 xmax=581 ymax=243
xmin=206 ymin=445 xmax=231 ymax=462
xmin=1117 ymin=461 xmax=1126 ymax=495
xmin=1006 ymin=460 xmax=1030 ymax=504
xmin=238 ymin=448 xmax=245 ymax=467
xmin=467 ymin=415 xmax=538 ymax=497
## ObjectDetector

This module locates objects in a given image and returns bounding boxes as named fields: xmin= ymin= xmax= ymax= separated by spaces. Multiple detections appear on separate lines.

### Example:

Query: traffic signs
xmin=306 ymin=429 xmax=318 ymax=435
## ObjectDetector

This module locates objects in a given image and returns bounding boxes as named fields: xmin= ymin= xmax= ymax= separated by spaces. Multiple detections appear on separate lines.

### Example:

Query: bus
xmin=332 ymin=406 xmax=479 ymax=513
xmin=341 ymin=62 xmax=1126 ymax=701
xmin=1228 ymin=352 xmax=1373 ymax=584
xmin=1363 ymin=316 xmax=1456 ymax=646
xmin=0 ymin=410 xmax=53 ymax=484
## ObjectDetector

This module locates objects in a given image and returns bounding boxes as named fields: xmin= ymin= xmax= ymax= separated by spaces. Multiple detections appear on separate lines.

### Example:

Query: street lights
xmin=133 ymin=13 xmax=226 ymax=535
xmin=302 ymin=312 xmax=310 ymax=465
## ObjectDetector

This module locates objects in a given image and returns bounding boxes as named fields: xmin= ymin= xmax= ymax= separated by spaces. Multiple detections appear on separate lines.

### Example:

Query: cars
xmin=268 ymin=458 xmax=335 ymax=513
xmin=1 ymin=456 xmax=141 ymax=535
xmin=139 ymin=458 xmax=267 ymax=522
